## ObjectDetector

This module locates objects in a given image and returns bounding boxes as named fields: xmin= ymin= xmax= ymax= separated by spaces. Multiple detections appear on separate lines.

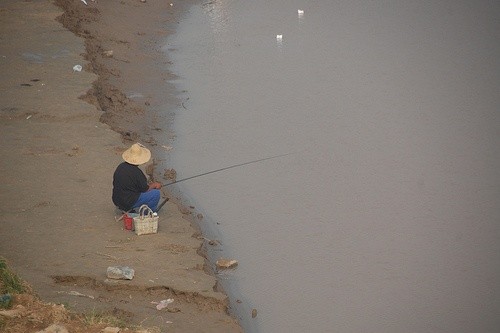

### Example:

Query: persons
xmin=112 ymin=142 xmax=162 ymax=218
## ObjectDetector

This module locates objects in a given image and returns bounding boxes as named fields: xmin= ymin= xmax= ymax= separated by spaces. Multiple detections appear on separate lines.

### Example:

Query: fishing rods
xmin=161 ymin=151 xmax=310 ymax=188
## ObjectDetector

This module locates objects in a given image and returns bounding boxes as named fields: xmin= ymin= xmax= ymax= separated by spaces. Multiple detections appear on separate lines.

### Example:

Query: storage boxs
xmin=132 ymin=204 xmax=159 ymax=235
xmin=123 ymin=212 xmax=139 ymax=230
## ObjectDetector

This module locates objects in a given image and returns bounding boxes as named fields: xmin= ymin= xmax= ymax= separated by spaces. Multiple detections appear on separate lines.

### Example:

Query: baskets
xmin=133 ymin=204 xmax=159 ymax=235
xmin=123 ymin=213 xmax=137 ymax=230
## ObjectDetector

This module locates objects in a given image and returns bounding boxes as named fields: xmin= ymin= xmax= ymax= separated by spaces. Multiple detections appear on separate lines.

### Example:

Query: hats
xmin=122 ymin=143 xmax=151 ymax=165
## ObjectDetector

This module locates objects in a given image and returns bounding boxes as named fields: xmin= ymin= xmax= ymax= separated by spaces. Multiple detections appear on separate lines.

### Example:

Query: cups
xmin=124 ymin=216 xmax=132 ymax=230
